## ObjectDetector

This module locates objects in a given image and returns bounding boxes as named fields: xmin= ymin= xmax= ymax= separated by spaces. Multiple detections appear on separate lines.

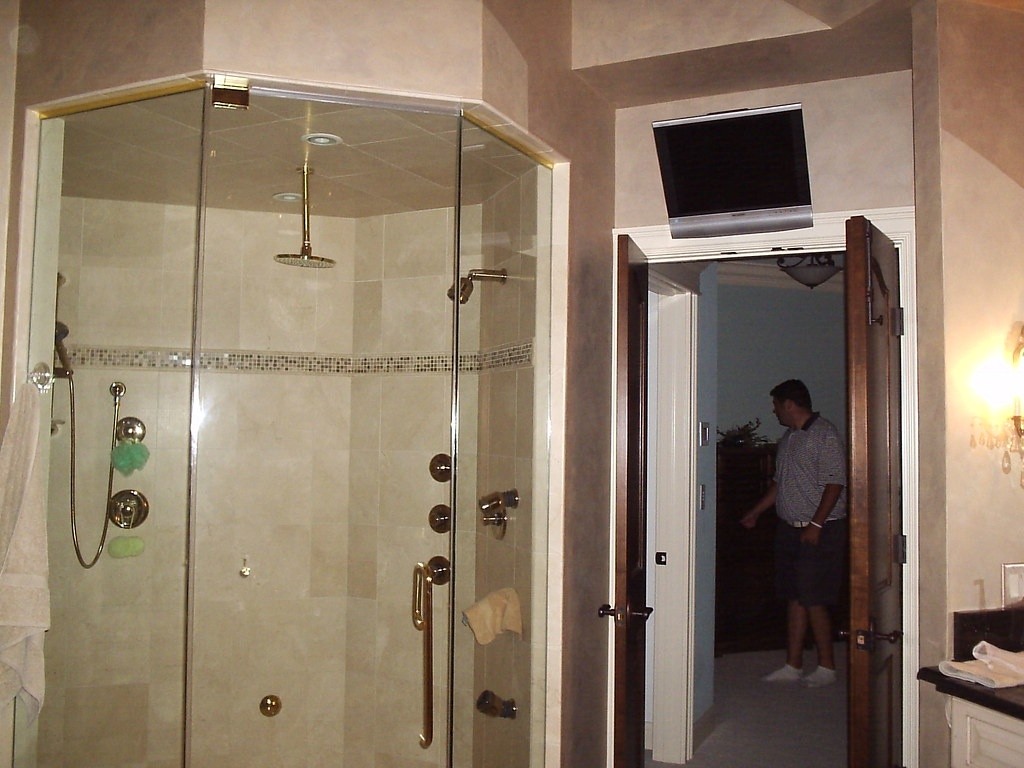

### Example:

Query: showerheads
xmin=448 ymin=279 xmax=472 ymax=307
xmin=55 ymin=322 xmax=74 ymax=375
xmin=270 ymin=244 xmax=336 ymax=270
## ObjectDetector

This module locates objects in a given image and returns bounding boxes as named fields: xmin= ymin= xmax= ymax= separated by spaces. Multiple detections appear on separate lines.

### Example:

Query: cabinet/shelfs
xmin=916 ymin=660 xmax=1024 ymax=768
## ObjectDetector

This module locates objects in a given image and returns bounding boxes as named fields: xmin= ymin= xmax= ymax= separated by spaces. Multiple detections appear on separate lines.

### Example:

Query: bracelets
xmin=809 ymin=521 xmax=823 ymax=528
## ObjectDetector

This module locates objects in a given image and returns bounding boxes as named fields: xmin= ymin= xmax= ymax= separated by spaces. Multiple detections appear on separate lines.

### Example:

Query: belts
xmin=788 ymin=517 xmax=837 ymax=527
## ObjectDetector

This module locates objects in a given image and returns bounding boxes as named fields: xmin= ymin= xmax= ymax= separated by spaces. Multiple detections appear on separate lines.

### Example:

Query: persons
xmin=741 ymin=378 xmax=849 ymax=689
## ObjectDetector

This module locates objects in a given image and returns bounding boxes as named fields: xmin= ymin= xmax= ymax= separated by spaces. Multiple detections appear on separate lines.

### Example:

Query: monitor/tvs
xmin=650 ymin=101 xmax=814 ymax=239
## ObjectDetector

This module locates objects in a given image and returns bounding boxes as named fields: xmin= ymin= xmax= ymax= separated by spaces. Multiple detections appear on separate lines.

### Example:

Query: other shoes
xmin=767 ymin=664 xmax=803 ymax=680
xmin=800 ymin=665 xmax=837 ymax=688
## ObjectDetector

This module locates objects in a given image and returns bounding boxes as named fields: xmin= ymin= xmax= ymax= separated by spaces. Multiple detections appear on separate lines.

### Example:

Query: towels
xmin=937 ymin=640 xmax=1024 ymax=690
xmin=0 ymin=379 xmax=52 ymax=729
xmin=461 ymin=588 xmax=521 ymax=646
